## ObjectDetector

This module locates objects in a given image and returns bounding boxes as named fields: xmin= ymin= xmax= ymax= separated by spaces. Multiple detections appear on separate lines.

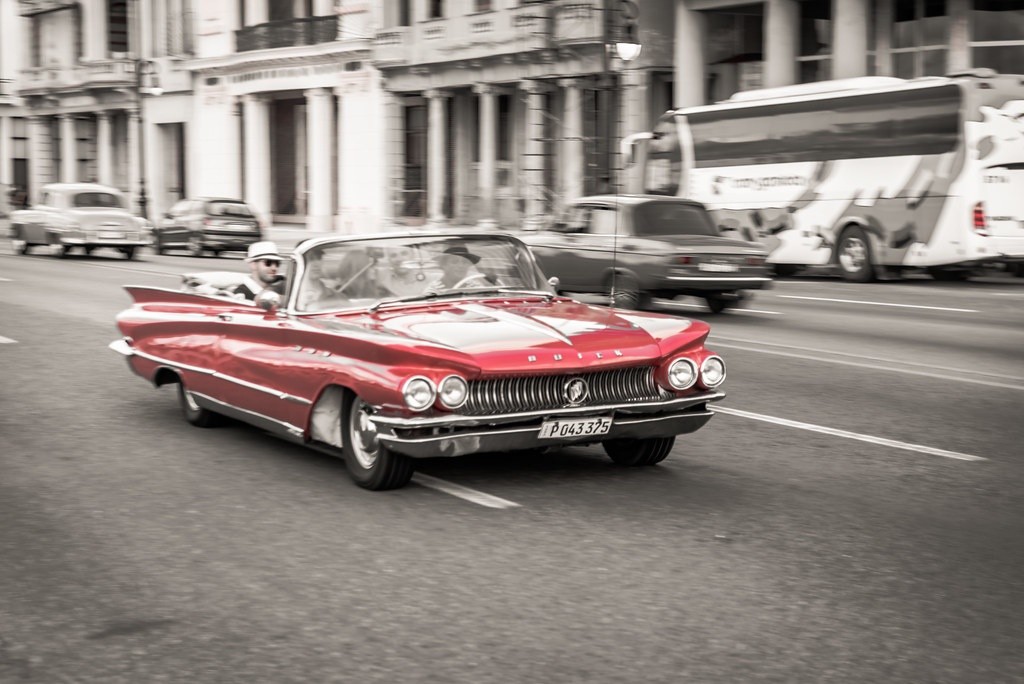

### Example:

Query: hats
xmin=431 ymin=240 xmax=481 ymax=267
xmin=244 ymin=241 xmax=288 ymax=262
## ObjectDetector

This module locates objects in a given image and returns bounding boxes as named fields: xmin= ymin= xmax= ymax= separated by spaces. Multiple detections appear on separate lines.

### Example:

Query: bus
xmin=618 ymin=67 xmax=1024 ymax=286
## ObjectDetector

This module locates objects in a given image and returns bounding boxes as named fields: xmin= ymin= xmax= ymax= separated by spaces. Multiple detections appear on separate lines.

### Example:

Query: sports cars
xmin=109 ymin=222 xmax=726 ymax=488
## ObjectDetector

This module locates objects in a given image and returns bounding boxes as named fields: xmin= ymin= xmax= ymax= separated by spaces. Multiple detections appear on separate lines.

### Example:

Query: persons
xmin=295 ymin=239 xmax=349 ymax=303
xmin=233 ymin=242 xmax=286 ymax=310
xmin=386 ymin=244 xmax=431 ymax=297
xmin=420 ymin=243 xmax=499 ymax=294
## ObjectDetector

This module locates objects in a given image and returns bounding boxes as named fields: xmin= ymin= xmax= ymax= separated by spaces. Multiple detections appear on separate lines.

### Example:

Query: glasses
xmin=450 ymin=257 xmax=469 ymax=265
xmin=253 ymin=259 xmax=279 ymax=267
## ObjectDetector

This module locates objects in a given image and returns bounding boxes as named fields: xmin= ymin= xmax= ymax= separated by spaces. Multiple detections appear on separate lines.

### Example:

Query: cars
xmin=152 ymin=196 xmax=263 ymax=258
xmin=7 ymin=182 xmax=155 ymax=260
xmin=520 ymin=193 xmax=770 ymax=316
